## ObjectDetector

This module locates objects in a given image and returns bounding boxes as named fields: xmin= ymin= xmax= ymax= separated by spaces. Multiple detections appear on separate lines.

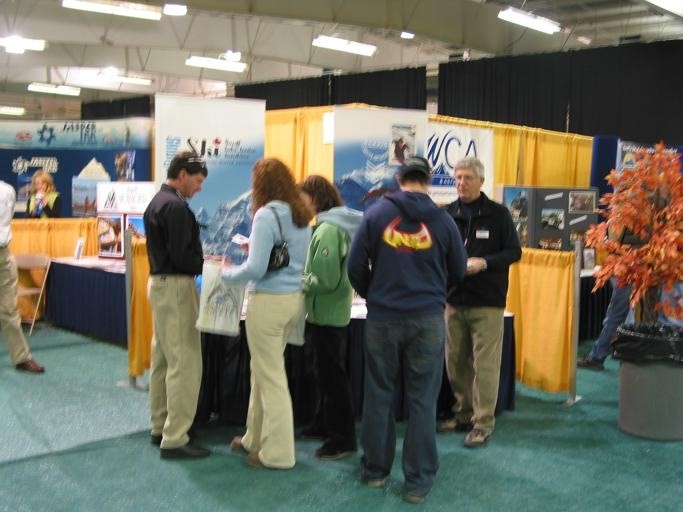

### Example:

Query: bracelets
xmin=481 ymin=258 xmax=487 ymax=272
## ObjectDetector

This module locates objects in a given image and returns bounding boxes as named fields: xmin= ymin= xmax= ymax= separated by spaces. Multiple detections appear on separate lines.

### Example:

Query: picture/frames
xmin=124 ymin=213 xmax=146 ymax=252
xmin=96 ymin=212 xmax=126 ymax=259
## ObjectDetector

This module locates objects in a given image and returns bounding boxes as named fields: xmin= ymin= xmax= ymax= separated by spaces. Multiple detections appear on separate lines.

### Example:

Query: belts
xmin=0 ymin=240 xmax=11 ymax=249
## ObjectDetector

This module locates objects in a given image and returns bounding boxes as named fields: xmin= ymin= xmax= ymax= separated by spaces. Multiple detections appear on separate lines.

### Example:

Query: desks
xmin=46 ymin=255 xmax=128 ymax=349
xmin=342 ymin=298 xmax=516 ymax=427
xmin=9 ymin=217 xmax=99 ymax=324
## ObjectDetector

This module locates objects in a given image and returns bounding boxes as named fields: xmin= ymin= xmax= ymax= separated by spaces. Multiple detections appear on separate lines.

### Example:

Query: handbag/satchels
xmin=268 ymin=206 xmax=290 ymax=272
xmin=194 ymin=254 xmax=247 ymax=338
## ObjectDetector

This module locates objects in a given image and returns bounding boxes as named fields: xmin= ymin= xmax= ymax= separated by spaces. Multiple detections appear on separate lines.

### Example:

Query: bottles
xmin=34 ymin=192 xmax=44 ymax=216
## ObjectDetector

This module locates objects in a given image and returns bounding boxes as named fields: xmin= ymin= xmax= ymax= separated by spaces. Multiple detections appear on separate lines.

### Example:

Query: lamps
xmin=497 ymin=3 xmax=566 ymax=36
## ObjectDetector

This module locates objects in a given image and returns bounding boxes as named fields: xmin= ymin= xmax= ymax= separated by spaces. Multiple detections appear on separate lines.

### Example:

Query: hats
xmin=399 ymin=155 xmax=431 ymax=179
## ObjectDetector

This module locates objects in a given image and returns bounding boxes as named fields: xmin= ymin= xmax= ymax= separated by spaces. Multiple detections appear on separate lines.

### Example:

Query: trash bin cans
xmin=615 ymin=323 xmax=683 ymax=441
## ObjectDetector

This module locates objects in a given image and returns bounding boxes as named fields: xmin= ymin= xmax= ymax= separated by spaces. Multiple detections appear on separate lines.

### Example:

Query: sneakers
xmin=245 ymin=451 xmax=296 ymax=471
xmin=436 ymin=414 xmax=474 ymax=433
xmin=360 ymin=469 xmax=385 ymax=488
xmin=230 ymin=435 xmax=243 ymax=451
xmin=463 ymin=428 xmax=495 ymax=448
xmin=401 ymin=488 xmax=424 ymax=504
xmin=295 ymin=426 xmax=324 ymax=441
xmin=315 ymin=442 xmax=352 ymax=462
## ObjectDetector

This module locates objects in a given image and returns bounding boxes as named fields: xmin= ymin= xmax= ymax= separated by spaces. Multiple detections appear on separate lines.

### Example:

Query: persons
xmin=218 ymin=156 xmax=313 ymax=473
xmin=0 ymin=177 xmax=45 ymax=375
xmin=392 ymin=137 xmax=409 ymax=164
xmin=344 ymin=156 xmax=468 ymax=503
xmin=142 ymin=152 xmax=211 ymax=462
xmin=297 ymin=173 xmax=359 ymax=462
xmin=576 ymin=277 xmax=634 ymax=372
xmin=433 ymin=157 xmax=522 ymax=450
xmin=24 ymin=169 xmax=61 ymax=216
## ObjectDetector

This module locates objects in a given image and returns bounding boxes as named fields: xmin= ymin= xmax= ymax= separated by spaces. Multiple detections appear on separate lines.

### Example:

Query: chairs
xmin=17 ymin=250 xmax=52 ymax=335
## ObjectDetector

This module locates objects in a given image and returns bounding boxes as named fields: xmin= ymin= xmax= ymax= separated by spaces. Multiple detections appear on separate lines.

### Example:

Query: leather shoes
xmin=150 ymin=434 xmax=163 ymax=444
xmin=16 ymin=359 xmax=45 ymax=373
xmin=577 ymin=354 xmax=604 ymax=371
xmin=159 ymin=439 xmax=211 ymax=459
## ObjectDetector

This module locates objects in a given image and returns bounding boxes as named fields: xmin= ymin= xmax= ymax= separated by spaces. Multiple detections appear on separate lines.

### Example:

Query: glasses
xmin=178 ymin=157 xmax=207 ymax=169
